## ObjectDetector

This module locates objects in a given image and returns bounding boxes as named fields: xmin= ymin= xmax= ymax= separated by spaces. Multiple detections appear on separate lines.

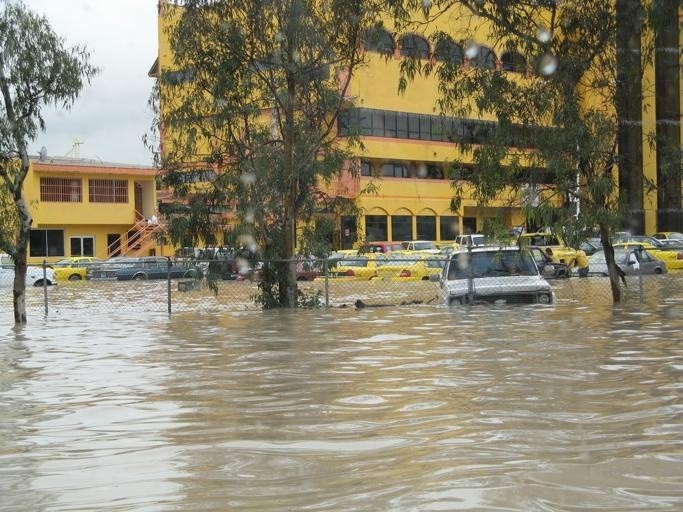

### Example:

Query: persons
xmin=543 ymin=248 xmax=560 ymax=265
xmin=566 ymin=247 xmax=589 ymax=277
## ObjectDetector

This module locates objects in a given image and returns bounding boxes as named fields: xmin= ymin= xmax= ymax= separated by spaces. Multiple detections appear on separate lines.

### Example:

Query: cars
xmin=84 ymin=255 xmax=203 ymax=281
xmin=52 ymin=256 xmax=104 ymax=281
xmin=2 ymin=252 xmax=57 ymax=289
xmin=428 ymin=246 xmax=556 ymax=308
xmin=171 ymin=223 xmax=680 ymax=284
